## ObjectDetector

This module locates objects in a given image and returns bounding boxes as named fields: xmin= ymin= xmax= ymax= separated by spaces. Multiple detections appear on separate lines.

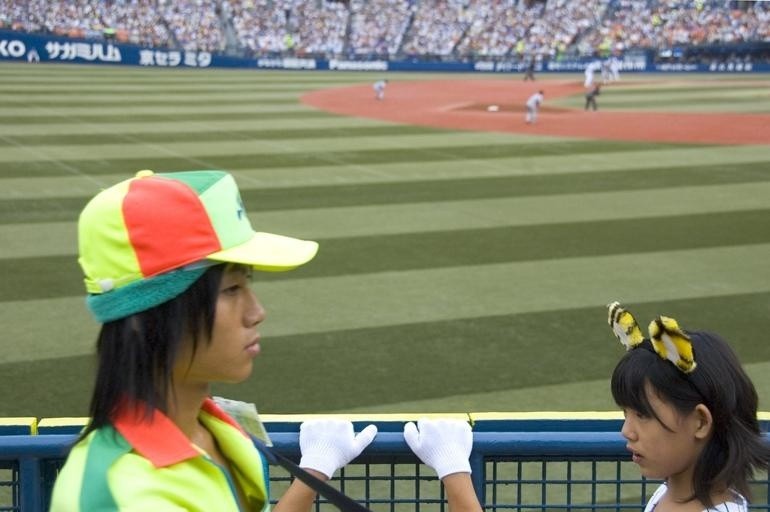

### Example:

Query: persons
xmin=2 ymin=1 xmax=770 ymax=65
xmin=521 ymin=55 xmax=535 ymax=81
xmin=402 ymin=298 xmax=770 ymax=510
xmin=374 ymin=79 xmax=390 ymax=100
xmin=601 ymin=55 xmax=620 ymax=82
xmin=585 ymin=86 xmax=600 ymax=111
xmin=525 ymin=90 xmax=545 ymax=123
xmin=583 ymin=59 xmax=603 ymax=88
xmin=41 ymin=167 xmax=379 ymax=511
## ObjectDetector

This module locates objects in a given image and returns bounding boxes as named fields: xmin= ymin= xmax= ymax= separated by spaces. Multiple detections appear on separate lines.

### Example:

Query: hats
xmin=76 ymin=167 xmax=320 ymax=301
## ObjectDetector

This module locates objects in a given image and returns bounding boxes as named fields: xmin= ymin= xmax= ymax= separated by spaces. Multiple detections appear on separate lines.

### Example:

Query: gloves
xmin=296 ymin=416 xmax=379 ymax=482
xmin=403 ymin=416 xmax=474 ymax=483
